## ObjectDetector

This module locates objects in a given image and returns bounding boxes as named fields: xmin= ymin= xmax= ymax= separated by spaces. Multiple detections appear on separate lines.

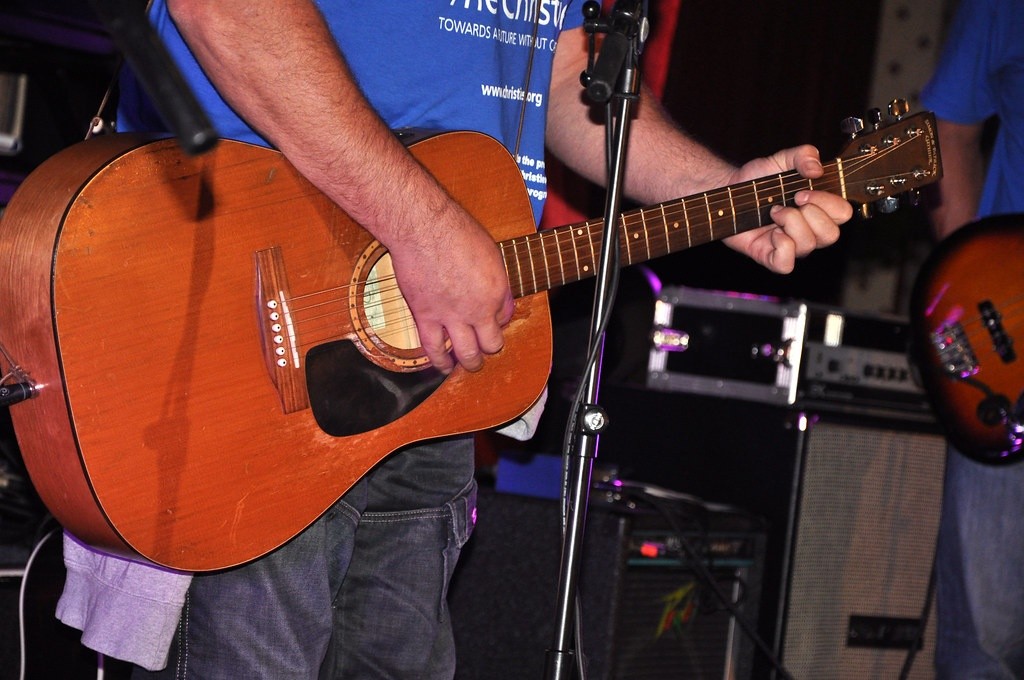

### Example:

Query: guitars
xmin=906 ymin=213 xmax=1024 ymax=467
xmin=1 ymin=99 xmax=945 ymax=577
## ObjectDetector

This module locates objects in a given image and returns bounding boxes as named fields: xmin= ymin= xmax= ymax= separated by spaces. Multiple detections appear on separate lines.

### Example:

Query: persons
xmin=109 ymin=0 xmax=853 ymax=679
xmin=926 ymin=0 xmax=1023 ymax=680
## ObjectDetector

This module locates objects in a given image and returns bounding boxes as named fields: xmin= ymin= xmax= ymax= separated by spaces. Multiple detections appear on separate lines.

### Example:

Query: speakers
xmin=761 ymin=405 xmax=947 ymax=680
xmin=447 ymin=475 xmax=766 ymax=680
